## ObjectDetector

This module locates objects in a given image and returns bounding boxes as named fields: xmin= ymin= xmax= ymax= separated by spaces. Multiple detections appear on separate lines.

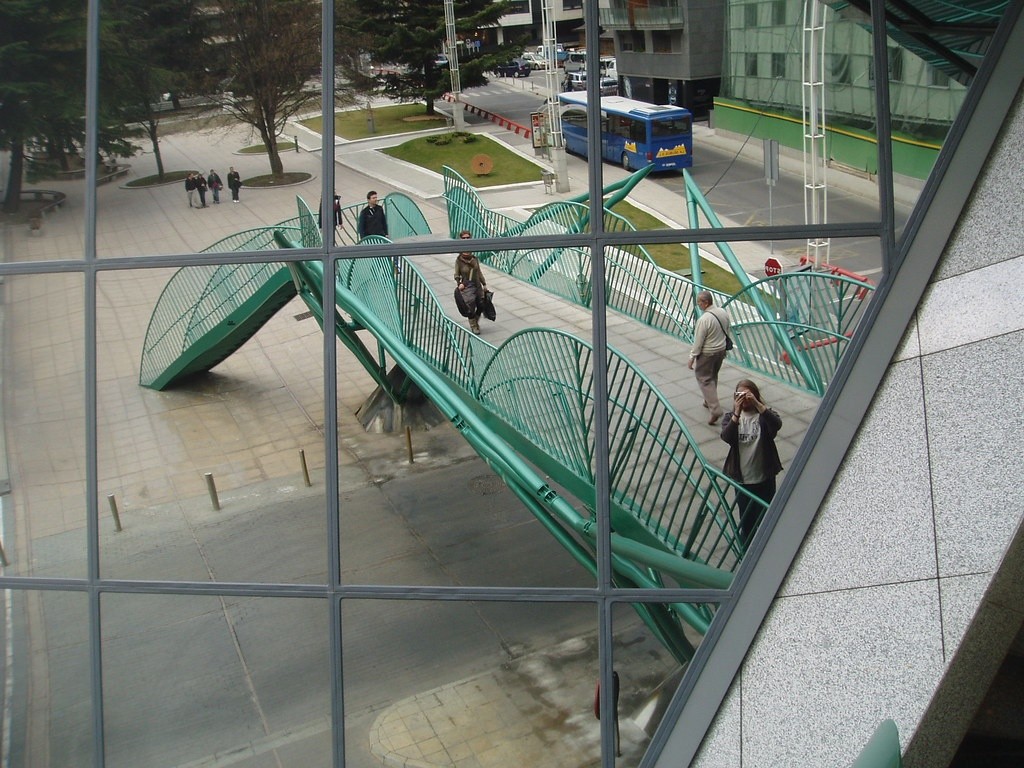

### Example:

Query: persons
xmin=185 ymin=166 xmax=240 ymax=208
xmin=359 ymin=190 xmax=389 ymax=243
xmin=319 ymin=189 xmax=344 ymax=231
xmin=563 ymin=77 xmax=572 ymax=92
xmin=688 ymin=291 xmax=730 ymax=425
xmin=719 ymin=379 xmax=783 ymax=555
xmin=454 ymin=231 xmax=487 ymax=335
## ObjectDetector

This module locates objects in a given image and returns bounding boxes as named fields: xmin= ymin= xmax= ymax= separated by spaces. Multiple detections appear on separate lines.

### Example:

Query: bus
xmin=538 ymin=88 xmax=694 ymax=174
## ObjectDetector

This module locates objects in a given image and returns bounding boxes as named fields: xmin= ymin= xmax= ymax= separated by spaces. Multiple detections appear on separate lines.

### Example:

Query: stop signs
xmin=764 ymin=257 xmax=783 ymax=281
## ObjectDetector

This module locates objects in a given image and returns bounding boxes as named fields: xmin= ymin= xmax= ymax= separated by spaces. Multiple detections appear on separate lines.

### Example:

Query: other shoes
xmin=216 ymin=200 xmax=219 ymax=204
xmin=232 ymin=200 xmax=237 ymax=203
xmin=469 ymin=319 xmax=481 ymax=335
xmin=237 ymin=200 xmax=240 ymax=203
xmin=703 ymin=399 xmax=708 ymax=407
xmin=708 ymin=411 xmax=724 ymax=424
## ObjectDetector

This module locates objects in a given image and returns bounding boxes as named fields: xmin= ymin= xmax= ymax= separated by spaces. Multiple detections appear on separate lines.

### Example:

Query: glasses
xmin=460 ymin=236 xmax=470 ymax=239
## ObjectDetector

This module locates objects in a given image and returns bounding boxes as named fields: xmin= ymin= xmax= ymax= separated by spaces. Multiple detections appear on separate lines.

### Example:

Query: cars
xmin=433 ymin=53 xmax=449 ymax=72
xmin=492 ymin=41 xmax=618 ymax=96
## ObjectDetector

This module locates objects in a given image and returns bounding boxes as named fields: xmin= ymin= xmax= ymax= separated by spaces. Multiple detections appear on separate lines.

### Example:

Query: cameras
xmin=734 ymin=392 xmax=745 ymax=399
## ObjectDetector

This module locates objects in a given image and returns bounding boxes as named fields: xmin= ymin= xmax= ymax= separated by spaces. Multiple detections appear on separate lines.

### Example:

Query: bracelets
xmin=733 ymin=412 xmax=740 ymax=419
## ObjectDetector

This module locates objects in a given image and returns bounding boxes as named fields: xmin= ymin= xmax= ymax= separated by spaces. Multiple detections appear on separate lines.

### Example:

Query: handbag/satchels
xmin=481 ymin=290 xmax=497 ymax=321
xmin=204 ymin=186 xmax=208 ymax=191
xmin=234 ymin=180 xmax=242 ymax=189
xmin=725 ymin=336 xmax=734 ymax=350
xmin=217 ymin=182 xmax=223 ymax=190
